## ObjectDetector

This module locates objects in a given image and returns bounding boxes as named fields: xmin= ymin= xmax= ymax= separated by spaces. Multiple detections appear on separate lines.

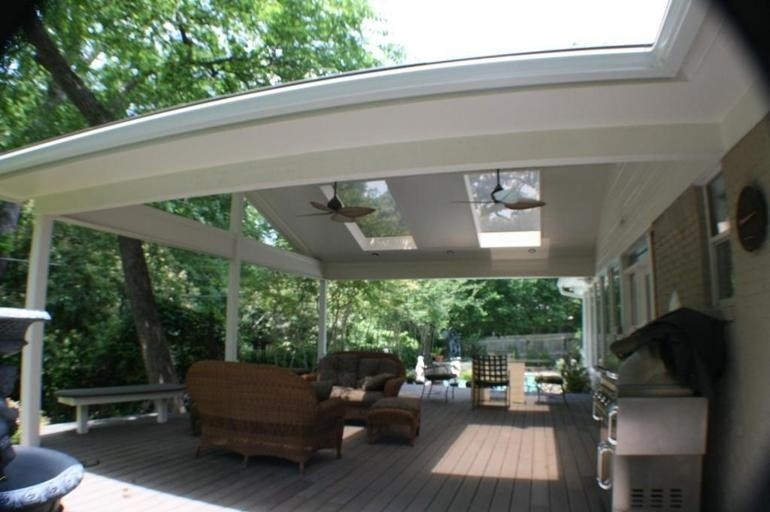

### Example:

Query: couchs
xmin=301 ymin=352 xmax=405 ymax=428
xmin=187 ymin=360 xmax=347 ymax=473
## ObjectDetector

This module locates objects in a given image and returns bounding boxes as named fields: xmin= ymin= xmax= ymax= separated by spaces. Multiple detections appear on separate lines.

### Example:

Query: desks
xmin=478 ymin=362 xmax=525 ymax=405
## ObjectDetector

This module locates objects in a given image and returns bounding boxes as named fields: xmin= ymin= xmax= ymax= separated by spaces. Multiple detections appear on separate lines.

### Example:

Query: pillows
xmin=307 ymin=353 xmax=399 ymax=400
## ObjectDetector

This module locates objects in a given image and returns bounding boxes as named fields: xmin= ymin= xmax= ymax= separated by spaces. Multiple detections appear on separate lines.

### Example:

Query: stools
xmin=367 ymin=397 xmax=421 ymax=446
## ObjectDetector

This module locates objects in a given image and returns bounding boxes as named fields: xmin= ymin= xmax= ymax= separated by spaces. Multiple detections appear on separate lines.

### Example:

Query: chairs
xmin=534 ymin=353 xmax=572 ymax=409
xmin=471 ymin=353 xmax=511 ymax=412
xmin=419 ymin=354 xmax=457 ymax=403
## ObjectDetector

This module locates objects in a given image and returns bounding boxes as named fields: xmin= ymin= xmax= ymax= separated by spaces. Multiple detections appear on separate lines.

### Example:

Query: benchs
xmin=54 ymin=383 xmax=187 ymax=434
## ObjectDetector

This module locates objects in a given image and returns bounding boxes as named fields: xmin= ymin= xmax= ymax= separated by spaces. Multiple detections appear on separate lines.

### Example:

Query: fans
xmin=453 ymin=169 xmax=546 ymax=218
xmin=295 ymin=182 xmax=376 ymax=223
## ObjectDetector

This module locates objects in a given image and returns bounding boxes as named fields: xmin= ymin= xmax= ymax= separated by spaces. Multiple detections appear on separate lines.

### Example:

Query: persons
xmin=415 ymin=355 xmax=426 ymax=384
